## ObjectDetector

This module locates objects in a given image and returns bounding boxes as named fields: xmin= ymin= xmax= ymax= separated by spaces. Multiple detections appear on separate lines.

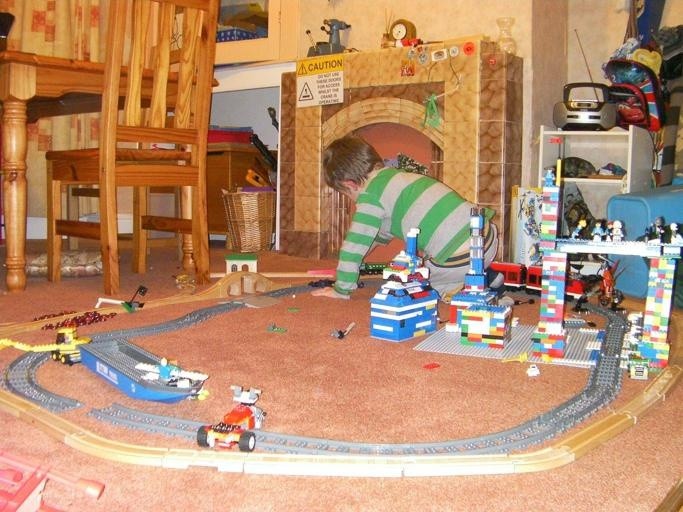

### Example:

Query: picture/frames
xmin=217 ymin=3 xmax=300 ymax=66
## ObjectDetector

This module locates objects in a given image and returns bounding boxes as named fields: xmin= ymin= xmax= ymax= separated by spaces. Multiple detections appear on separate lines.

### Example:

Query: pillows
xmin=23 ymin=247 xmax=118 ymax=276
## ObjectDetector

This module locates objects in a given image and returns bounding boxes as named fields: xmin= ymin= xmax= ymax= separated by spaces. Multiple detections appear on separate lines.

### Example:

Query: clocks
xmin=391 ymin=19 xmax=416 ymax=44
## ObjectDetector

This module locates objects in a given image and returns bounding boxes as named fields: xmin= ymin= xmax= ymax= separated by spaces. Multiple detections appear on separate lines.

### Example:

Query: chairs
xmin=45 ymin=3 xmax=222 ymax=294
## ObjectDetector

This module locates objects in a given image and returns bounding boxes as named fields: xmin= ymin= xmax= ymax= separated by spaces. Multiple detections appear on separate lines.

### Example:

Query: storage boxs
xmin=608 ymin=175 xmax=683 ymax=304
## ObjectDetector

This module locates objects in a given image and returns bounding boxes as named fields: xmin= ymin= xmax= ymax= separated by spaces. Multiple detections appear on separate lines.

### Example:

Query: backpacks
xmin=602 ymin=0 xmax=672 ymax=131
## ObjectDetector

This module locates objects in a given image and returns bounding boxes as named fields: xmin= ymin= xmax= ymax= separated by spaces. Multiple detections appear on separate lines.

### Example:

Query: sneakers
xmin=485 ymin=268 xmax=507 ymax=290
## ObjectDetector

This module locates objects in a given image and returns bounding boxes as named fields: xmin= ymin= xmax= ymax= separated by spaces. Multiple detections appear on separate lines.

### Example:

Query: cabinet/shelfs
xmin=535 ymin=124 xmax=652 ymax=231
xmin=209 ymin=146 xmax=278 ymax=236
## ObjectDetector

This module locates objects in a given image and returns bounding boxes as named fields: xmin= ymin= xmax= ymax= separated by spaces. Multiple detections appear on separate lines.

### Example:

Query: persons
xmin=305 ymin=131 xmax=499 ymax=298
